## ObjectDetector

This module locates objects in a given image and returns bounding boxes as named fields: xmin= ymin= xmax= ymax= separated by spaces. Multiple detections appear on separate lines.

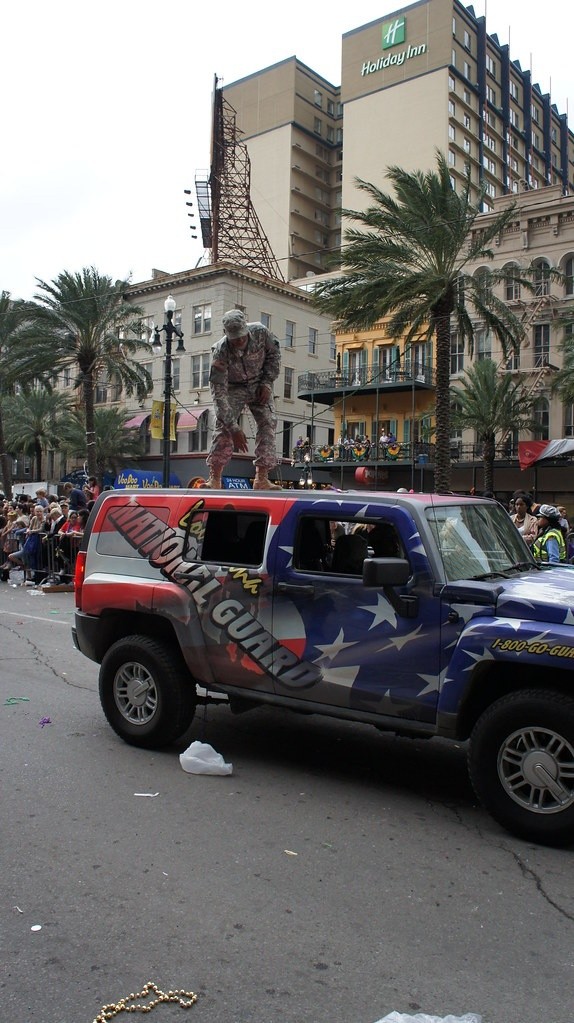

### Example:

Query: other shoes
xmin=59 ymin=569 xmax=64 ymax=576
xmin=0 ymin=561 xmax=12 ymax=571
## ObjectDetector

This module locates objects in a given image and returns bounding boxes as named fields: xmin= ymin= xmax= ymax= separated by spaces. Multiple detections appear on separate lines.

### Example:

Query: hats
xmin=536 ymin=504 xmax=562 ymax=520
xmin=223 ymin=309 xmax=248 ymax=339
xmin=60 ymin=503 xmax=68 ymax=507
xmin=7 ymin=512 xmax=16 ymax=516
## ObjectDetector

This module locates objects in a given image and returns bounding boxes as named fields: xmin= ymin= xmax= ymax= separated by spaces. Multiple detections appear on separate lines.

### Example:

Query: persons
xmin=330 ymin=520 xmax=374 ymax=549
xmin=379 ymin=431 xmax=400 ymax=461
xmin=205 ymin=309 xmax=286 ymax=491
xmin=438 ymin=488 xmax=570 ymax=563
xmin=0 ymin=476 xmax=100 ymax=586
xmin=338 ymin=435 xmax=371 ymax=461
xmin=296 ymin=435 xmax=311 ymax=461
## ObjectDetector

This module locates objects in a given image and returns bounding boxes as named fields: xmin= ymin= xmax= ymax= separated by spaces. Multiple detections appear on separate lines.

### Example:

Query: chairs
xmin=332 ymin=535 xmax=368 ymax=575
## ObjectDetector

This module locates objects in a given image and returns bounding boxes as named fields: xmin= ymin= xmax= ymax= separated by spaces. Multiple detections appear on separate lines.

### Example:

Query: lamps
xmin=193 ymin=391 xmax=199 ymax=404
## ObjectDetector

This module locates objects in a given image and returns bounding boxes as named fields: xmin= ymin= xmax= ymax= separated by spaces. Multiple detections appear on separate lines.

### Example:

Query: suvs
xmin=71 ymin=488 xmax=574 ymax=843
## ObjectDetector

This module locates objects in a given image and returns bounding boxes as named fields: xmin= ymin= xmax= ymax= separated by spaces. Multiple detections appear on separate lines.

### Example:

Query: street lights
xmin=300 ymin=455 xmax=313 ymax=489
xmin=152 ymin=295 xmax=186 ymax=488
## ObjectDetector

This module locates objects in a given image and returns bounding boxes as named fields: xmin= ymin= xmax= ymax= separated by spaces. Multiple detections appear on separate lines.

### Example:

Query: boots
xmin=199 ymin=466 xmax=223 ymax=489
xmin=252 ymin=466 xmax=282 ymax=490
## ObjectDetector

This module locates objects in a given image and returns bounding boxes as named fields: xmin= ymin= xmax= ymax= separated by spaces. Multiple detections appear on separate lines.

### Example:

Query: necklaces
xmin=516 ymin=517 xmax=525 ymax=523
